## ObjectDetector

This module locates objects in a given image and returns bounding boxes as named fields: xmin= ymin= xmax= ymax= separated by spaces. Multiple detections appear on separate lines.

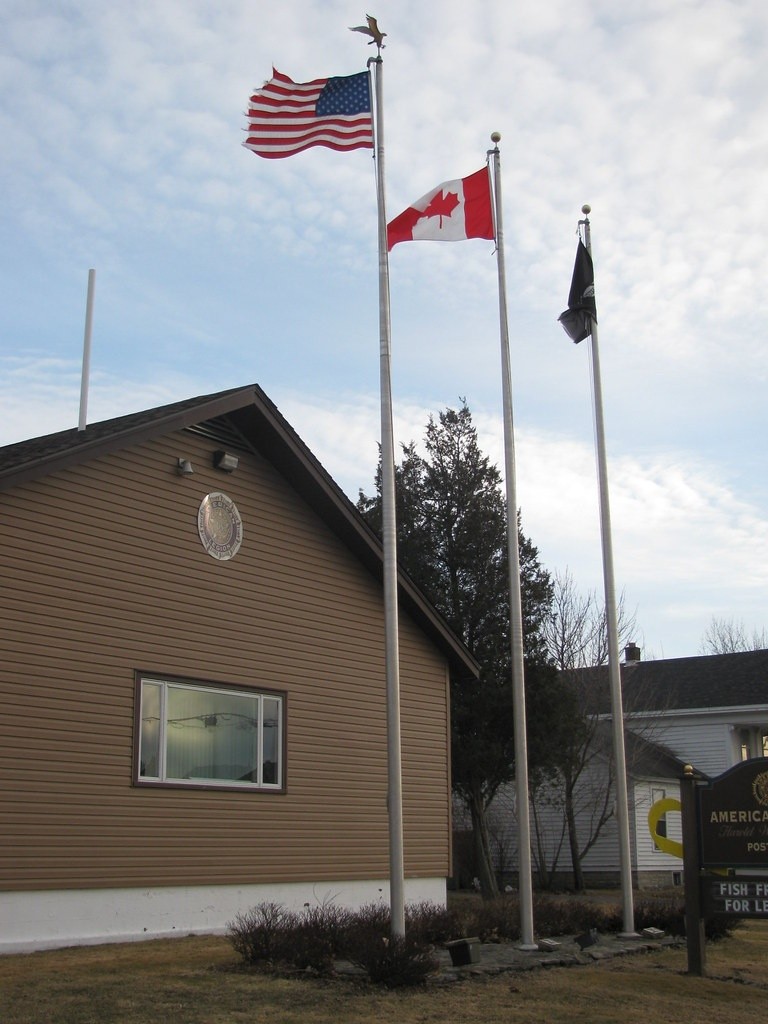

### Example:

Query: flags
xmin=382 ymin=167 xmax=497 ymax=251
xmin=555 ymin=241 xmax=600 ymax=344
xmin=242 ymin=63 xmax=374 ymax=159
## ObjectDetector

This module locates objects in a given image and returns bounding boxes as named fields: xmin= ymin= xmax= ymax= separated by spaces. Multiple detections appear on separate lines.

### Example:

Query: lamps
xmin=538 ymin=938 xmax=561 ymax=952
xmin=641 ymin=927 xmax=665 ymax=939
xmin=179 ymin=457 xmax=194 ymax=474
xmin=214 ymin=449 xmax=240 ymax=473
xmin=446 ymin=936 xmax=482 ymax=965
xmin=573 ymin=927 xmax=599 ymax=950
